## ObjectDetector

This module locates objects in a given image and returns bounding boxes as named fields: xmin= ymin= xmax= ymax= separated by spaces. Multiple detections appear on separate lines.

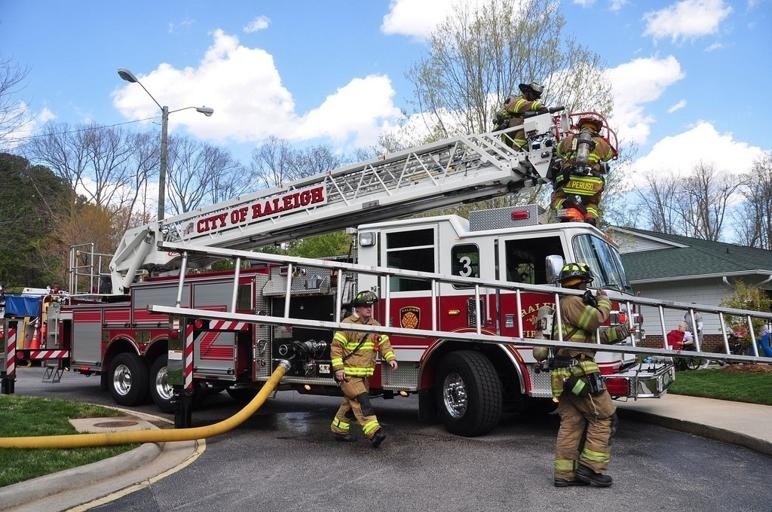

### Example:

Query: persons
xmin=492 ymin=82 xmax=549 ymax=152
xmin=329 ymin=291 xmax=398 ymax=446
xmin=551 ymin=263 xmax=637 ymax=486
xmin=684 ymin=302 xmax=710 ymax=368
xmin=677 ymin=322 xmax=693 ymax=350
xmin=750 ymin=324 xmax=772 ymax=357
xmin=556 ymin=117 xmax=615 ymax=226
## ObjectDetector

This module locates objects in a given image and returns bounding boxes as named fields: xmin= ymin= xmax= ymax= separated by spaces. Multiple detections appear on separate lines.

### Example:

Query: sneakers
xmin=555 ymin=464 xmax=613 ymax=487
xmin=371 ymin=431 xmax=386 ymax=448
xmin=334 ymin=431 xmax=354 ymax=442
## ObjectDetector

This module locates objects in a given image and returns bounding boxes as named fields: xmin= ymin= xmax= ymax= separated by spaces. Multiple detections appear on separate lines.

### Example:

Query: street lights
xmin=117 ymin=66 xmax=214 ymax=222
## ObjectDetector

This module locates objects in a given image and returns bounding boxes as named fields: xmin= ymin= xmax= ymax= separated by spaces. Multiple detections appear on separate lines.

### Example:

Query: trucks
xmin=20 ymin=286 xmax=70 ymax=299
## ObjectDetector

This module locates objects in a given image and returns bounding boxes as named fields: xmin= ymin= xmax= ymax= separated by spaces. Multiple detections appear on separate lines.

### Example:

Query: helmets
xmin=557 ymin=263 xmax=593 ymax=284
xmin=351 ymin=291 xmax=378 ymax=306
xmin=579 ymin=117 xmax=604 ymax=133
xmin=518 ymin=83 xmax=544 ymax=95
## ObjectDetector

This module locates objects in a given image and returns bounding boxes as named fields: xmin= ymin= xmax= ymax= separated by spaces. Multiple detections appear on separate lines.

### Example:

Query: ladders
xmin=146 ymin=240 xmax=772 ymax=363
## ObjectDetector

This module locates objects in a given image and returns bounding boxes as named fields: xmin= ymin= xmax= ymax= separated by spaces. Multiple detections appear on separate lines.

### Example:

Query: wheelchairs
xmin=721 ymin=334 xmax=756 ymax=366
xmin=671 ymin=341 xmax=701 ymax=373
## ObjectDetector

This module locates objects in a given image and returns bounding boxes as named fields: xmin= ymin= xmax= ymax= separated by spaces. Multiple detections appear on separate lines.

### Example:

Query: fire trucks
xmin=0 ymin=110 xmax=674 ymax=435
